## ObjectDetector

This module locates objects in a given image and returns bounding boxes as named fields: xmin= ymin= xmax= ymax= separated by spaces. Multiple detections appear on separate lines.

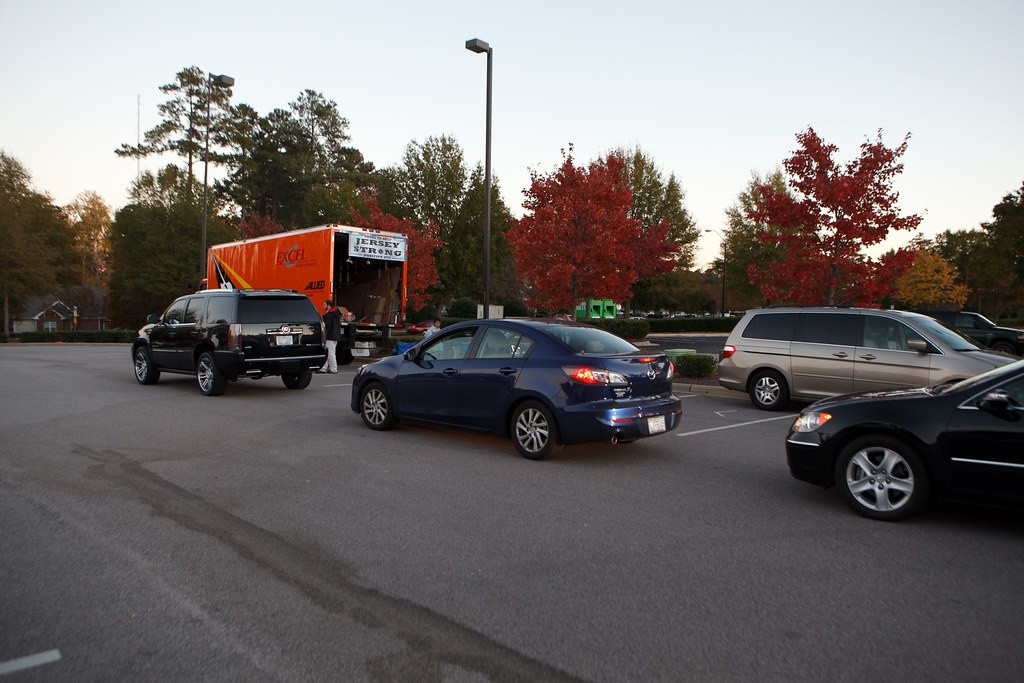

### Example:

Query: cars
xmin=406 ymin=320 xmax=434 ymax=336
xmin=349 ymin=318 xmax=684 ymax=460
xmin=784 ymin=357 xmax=1024 ymax=522
xmin=932 ymin=309 xmax=1024 ymax=359
xmin=616 ymin=310 xmax=712 ymax=317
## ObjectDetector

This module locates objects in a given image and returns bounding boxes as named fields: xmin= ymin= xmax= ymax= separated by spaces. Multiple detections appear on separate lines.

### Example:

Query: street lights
xmin=705 ymin=229 xmax=726 ymax=314
xmin=465 ymin=38 xmax=493 ymax=318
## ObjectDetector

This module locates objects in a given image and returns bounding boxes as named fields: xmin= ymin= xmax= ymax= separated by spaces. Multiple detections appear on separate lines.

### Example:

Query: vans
xmin=717 ymin=307 xmax=1019 ymax=411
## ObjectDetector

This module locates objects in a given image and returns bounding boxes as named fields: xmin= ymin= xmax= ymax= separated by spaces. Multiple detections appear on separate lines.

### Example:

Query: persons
xmin=314 ymin=300 xmax=342 ymax=374
xmin=423 ymin=317 xmax=446 ymax=361
xmin=555 ymin=309 xmax=574 ymax=345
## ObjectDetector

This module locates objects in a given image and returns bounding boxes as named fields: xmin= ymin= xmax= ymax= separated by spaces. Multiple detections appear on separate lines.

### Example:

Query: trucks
xmin=197 ymin=223 xmax=409 ymax=364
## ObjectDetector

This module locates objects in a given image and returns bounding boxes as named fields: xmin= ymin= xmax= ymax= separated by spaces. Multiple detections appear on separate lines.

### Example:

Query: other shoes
xmin=327 ymin=370 xmax=337 ymax=374
xmin=315 ymin=370 xmax=326 ymax=374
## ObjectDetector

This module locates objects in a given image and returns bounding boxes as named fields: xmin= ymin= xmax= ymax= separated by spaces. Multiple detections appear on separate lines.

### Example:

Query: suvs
xmin=131 ymin=288 xmax=329 ymax=397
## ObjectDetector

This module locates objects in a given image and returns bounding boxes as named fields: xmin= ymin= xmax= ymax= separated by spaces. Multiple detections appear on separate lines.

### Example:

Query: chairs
xmin=486 ymin=331 xmax=514 ymax=358
xmin=881 ymin=326 xmax=903 ymax=350
xmin=863 ymin=325 xmax=880 ymax=348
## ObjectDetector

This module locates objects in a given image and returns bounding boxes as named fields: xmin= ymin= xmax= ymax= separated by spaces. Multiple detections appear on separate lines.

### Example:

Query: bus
xmin=724 ymin=307 xmax=762 ymax=317
